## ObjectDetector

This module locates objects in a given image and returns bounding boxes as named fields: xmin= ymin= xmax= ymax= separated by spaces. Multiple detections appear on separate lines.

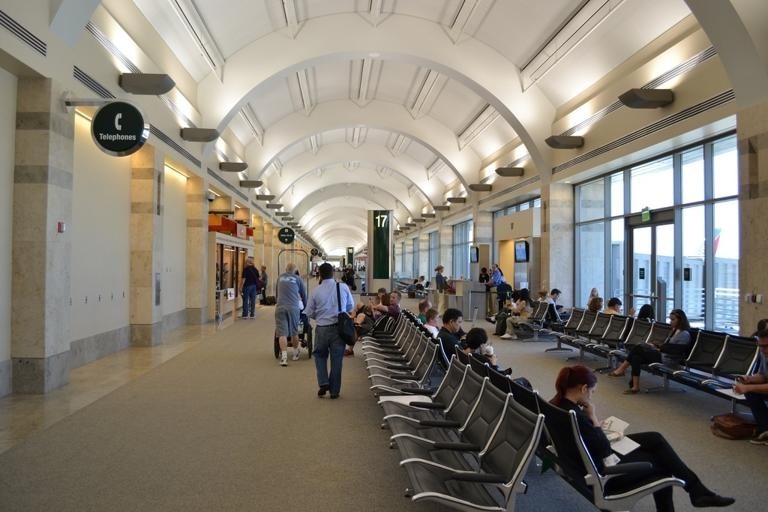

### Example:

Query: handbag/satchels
xmin=336 ymin=282 xmax=358 ymax=345
xmin=710 ymin=413 xmax=753 ymax=439
xmin=660 ymin=343 xmax=689 ymax=355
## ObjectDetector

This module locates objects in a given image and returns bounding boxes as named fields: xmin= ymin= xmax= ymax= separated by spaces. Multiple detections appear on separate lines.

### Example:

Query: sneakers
xmin=280 ymin=357 xmax=288 ymax=366
xmin=292 ymin=348 xmax=301 ymax=361
xmin=749 ymin=431 xmax=768 ymax=444
xmin=511 ymin=335 xmax=518 ymax=340
xmin=500 ymin=333 xmax=512 ymax=339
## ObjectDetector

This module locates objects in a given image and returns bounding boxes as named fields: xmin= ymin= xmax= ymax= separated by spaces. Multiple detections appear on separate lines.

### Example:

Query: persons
xmin=548 ymin=365 xmax=735 ymax=511
xmin=423 ymin=308 xmax=439 ymax=340
xmin=314 ymin=263 xmax=366 ymax=291
xmin=343 ymin=287 xmax=402 ymax=356
xmin=287 ymin=292 xmax=304 ymax=347
xmin=239 ymin=257 xmax=260 ymax=320
xmin=259 ymin=265 xmax=267 ymax=305
xmin=301 ymin=262 xmax=354 ymax=400
xmin=417 ymin=299 xmax=439 ymax=330
xmin=461 ymin=327 xmax=538 ymax=394
xmin=274 ymin=262 xmax=308 ymax=366
xmin=434 ymin=263 xmax=456 ymax=317
xmin=731 ymin=319 xmax=768 ymax=445
xmin=588 ymin=288 xmax=655 ymax=318
xmin=436 ymin=308 xmax=467 ymax=374
xmin=479 ymin=263 xmax=567 ymax=340
xmin=401 ymin=276 xmax=426 ymax=293
xmin=608 ymin=308 xmax=693 ymax=394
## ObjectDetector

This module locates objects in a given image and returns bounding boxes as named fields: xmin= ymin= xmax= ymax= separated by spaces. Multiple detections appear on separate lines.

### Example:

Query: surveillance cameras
xmin=208 ymin=192 xmax=215 ymax=202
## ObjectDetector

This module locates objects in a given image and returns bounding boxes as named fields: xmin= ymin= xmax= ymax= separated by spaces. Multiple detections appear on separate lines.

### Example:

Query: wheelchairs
xmin=274 ymin=310 xmax=313 ymax=359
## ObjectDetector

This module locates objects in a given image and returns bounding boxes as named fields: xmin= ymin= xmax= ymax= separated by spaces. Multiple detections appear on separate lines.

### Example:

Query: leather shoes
xmin=330 ymin=393 xmax=339 ymax=399
xmin=604 ymin=370 xmax=625 ymax=378
xmin=623 ymin=388 xmax=640 ymax=394
xmin=318 ymin=385 xmax=329 ymax=396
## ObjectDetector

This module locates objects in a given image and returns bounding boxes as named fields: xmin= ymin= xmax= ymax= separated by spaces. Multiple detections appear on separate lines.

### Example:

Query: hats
xmin=751 ymin=319 xmax=768 ymax=337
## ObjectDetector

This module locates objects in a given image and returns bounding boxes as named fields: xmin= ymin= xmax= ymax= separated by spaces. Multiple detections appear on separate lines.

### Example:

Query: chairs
xmin=394 ymin=278 xmax=428 ymax=297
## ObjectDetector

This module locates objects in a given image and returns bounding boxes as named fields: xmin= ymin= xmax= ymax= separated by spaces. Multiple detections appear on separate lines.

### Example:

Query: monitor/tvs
xmin=470 ymin=245 xmax=479 ymax=263
xmin=514 ymin=240 xmax=529 ymax=263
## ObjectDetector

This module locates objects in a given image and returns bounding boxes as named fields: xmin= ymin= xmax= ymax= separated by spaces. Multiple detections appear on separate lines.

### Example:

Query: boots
xmin=688 ymin=482 xmax=735 ymax=507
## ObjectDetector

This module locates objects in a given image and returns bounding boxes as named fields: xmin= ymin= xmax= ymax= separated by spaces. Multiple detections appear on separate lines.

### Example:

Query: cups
xmin=485 ymin=346 xmax=493 ymax=356
